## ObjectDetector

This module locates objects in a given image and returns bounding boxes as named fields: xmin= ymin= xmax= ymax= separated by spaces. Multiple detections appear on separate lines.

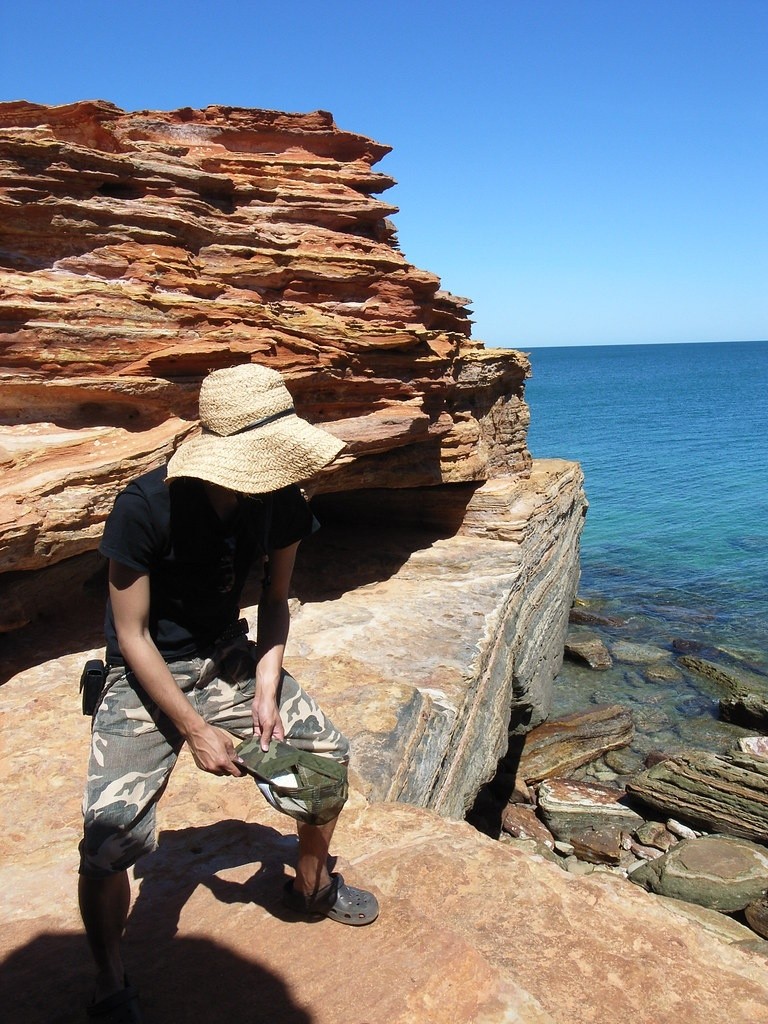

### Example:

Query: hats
xmin=235 ymin=736 xmax=347 ymax=825
xmin=164 ymin=364 xmax=346 ymax=496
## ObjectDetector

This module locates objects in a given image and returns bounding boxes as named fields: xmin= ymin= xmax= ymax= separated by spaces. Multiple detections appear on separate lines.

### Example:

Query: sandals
xmin=87 ymin=974 xmax=141 ymax=1024
xmin=279 ymin=873 xmax=380 ymax=926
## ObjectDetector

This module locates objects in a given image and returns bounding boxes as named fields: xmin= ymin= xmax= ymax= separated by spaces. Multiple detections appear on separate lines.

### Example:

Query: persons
xmin=79 ymin=361 xmax=379 ymax=1024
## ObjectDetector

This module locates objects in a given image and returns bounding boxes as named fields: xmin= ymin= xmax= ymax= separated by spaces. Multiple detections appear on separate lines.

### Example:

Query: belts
xmin=210 ymin=619 xmax=248 ymax=644
